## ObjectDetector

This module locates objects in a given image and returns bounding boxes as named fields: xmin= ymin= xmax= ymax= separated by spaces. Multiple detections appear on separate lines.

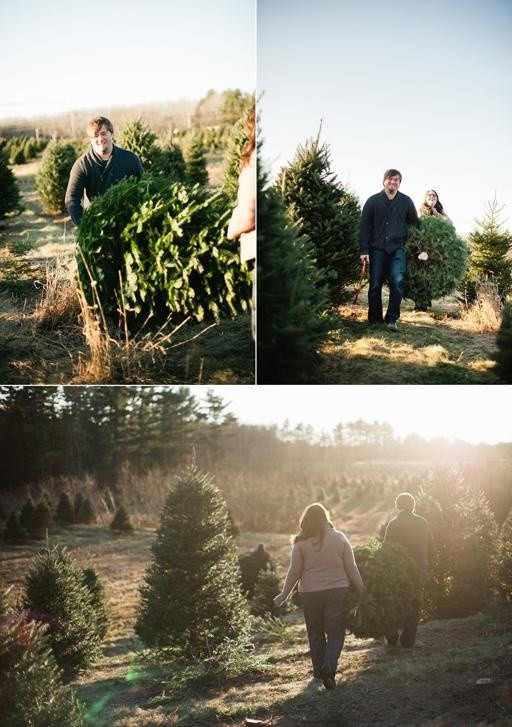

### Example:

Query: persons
xmin=382 ymin=492 xmax=435 ymax=651
xmin=359 ymin=168 xmax=421 ymax=332
xmin=63 ymin=115 xmax=148 ymax=340
xmin=272 ymin=503 xmax=365 ymax=690
xmin=226 ymin=102 xmax=257 ymax=378
xmin=411 ymin=187 xmax=449 ymax=312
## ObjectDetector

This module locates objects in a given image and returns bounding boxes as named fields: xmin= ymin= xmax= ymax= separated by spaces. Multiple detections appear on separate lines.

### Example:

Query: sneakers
xmin=386 ymin=322 xmax=399 ymax=331
xmin=313 ymin=671 xmax=337 ymax=689
